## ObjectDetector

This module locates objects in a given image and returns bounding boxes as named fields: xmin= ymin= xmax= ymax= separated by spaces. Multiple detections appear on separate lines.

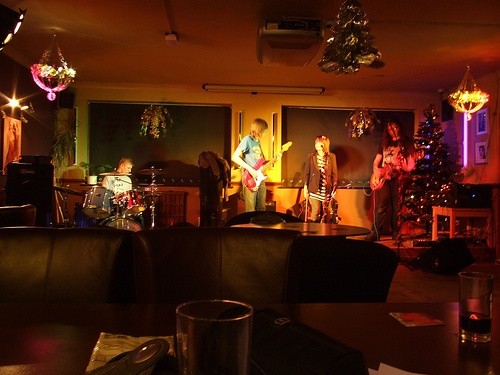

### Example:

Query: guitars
xmin=244 ymin=141 xmax=293 ymax=193
xmin=369 ymin=148 xmax=425 ymax=191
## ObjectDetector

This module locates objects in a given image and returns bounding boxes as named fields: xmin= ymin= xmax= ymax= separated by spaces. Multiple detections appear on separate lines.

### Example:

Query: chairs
xmin=0 ymin=226 xmax=135 ymax=305
xmin=135 ymin=227 xmax=300 ymax=309
xmin=290 ymin=236 xmax=399 ymax=303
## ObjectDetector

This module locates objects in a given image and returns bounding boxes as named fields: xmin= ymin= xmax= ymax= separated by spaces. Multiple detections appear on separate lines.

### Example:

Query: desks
xmin=228 ymin=220 xmax=371 ymax=240
xmin=431 ymin=206 xmax=492 ymax=245
xmin=0 ymin=302 xmax=500 ymax=375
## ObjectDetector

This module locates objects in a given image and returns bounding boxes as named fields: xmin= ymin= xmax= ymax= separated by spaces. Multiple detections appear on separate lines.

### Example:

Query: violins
xmin=322 ymin=180 xmax=340 ymax=224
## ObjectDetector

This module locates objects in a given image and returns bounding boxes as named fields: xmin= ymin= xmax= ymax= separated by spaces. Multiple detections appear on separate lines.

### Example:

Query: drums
xmin=81 ymin=187 xmax=115 ymax=220
xmin=116 ymin=188 xmax=149 ymax=217
xmin=97 ymin=215 xmax=143 ymax=232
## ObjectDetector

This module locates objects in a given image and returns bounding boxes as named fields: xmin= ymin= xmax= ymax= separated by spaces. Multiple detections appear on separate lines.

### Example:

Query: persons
xmin=372 ymin=118 xmax=415 ymax=235
xmin=477 ymin=144 xmax=486 ymax=161
xmin=230 ymin=118 xmax=283 ymax=213
xmin=303 ymin=134 xmax=339 ymax=223
xmin=101 ymin=156 xmax=136 ymax=205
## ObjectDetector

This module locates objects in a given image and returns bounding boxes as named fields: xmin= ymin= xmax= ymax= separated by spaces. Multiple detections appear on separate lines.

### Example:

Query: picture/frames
xmin=475 ymin=141 xmax=489 ymax=164
xmin=475 ymin=107 xmax=488 ymax=136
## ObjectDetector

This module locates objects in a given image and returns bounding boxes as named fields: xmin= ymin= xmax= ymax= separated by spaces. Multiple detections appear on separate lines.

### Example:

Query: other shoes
xmin=362 ymin=233 xmax=380 ymax=241
xmin=393 ymin=239 xmax=402 ymax=246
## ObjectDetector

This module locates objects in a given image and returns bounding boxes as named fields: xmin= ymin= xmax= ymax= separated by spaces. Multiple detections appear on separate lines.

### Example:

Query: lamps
xmin=448 ymin=65 xmax=490 ymax=121
xmin=31 ymin=30 xmax=76 ymax=101
xmin=203 ymin=83 xmax=324 ymax=96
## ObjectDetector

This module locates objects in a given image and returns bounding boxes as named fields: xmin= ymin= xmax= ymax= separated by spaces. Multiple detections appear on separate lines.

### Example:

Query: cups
xmin=458 ymin=271 xmax=494 ymax=343
xmin=174 ymin=299 xmax=254 ymax=375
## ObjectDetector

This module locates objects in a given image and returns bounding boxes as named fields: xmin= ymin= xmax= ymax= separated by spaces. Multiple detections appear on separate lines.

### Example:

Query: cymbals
xmin=138 ymin=184 xmax=166 ymax=186
xmin=98 ymin=173 xmax=132 ymax=176
xmin=136 ymin=168 xmax=171 ymax=175
xmin=48 ymin=186 xmax=84 ymax=196
